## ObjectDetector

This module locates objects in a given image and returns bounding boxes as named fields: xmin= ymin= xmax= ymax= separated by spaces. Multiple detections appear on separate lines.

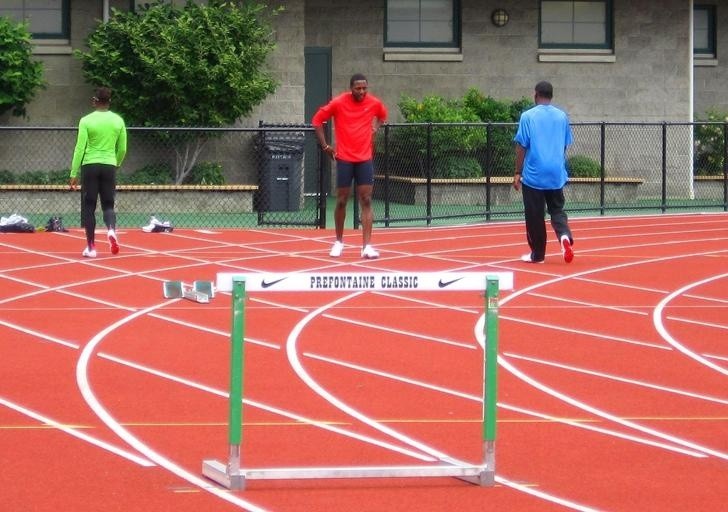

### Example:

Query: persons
xmin=511 ymin=80 xmax=575 ymax=264
xmin=67 ymin=86 xmax=128 ymax=259
xmin=311 ymin=73 xmax=387 ymax=259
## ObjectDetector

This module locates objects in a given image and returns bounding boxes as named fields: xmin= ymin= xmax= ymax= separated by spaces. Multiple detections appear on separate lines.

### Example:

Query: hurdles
xmin=201 ymin=269 xmax=514 ymax=491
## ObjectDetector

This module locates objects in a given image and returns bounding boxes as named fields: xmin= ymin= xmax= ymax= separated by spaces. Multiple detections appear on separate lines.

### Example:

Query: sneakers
xmin=521 ymin=253 xmax=545 ymax=264
xmin=561 ymin=235 xmax=574 ymax=263
xmin=329 ymin=241 xmax=344 ymax=256
xmin=108 ymin=230 xmax=120 ymax=254
xmin=82 ymin=247 xmax=96 ymax=257
xmin=361 ymin=243 xmax=379 ymax=258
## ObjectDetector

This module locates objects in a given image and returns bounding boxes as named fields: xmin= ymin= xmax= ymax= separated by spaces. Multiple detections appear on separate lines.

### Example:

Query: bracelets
xmin=514 ymin=172 xmax=522 ymax=175
xmin=323 ymin=144 xmax=329 ymax=152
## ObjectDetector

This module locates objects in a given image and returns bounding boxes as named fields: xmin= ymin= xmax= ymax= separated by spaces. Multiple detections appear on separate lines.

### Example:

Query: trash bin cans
xmin=252 ymin=131 xmax=306 ymax=213
